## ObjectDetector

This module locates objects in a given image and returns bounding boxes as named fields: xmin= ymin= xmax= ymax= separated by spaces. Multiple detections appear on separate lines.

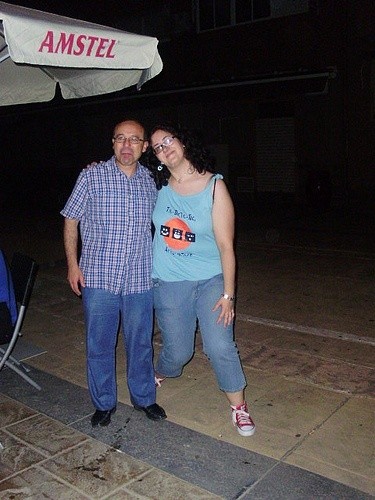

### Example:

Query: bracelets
xmin=220 ymin=294 xmax=234 ymax=301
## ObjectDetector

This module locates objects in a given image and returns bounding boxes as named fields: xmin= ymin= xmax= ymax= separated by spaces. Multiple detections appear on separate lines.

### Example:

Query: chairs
xmin=0 ymin=251 xmax=41 ymax=391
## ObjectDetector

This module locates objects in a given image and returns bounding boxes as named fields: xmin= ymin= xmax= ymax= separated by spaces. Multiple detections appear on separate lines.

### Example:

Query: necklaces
xmin=175 ymin=168 xmax=190 ymax=184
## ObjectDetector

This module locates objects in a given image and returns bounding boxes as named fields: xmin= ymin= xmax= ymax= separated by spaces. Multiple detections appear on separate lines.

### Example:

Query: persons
xmin=61 ymin=119 xmax=167 ymax=427
xmin=82 ymin=121 xmax=256 ymax=436
xmin=0 ymin=251 xmax=17 ymax=335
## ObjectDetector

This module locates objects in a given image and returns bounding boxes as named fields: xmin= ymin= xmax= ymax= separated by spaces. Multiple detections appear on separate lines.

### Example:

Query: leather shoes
xmin=91 ymin=404 xmax=117 ymax=428
xmin=134 ymin=403 xmax=167 ymax=421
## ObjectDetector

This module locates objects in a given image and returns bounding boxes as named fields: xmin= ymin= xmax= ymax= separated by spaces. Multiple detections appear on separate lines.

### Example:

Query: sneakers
xmin=155 ymin=371 xmax=166 ymax=388
xmin=231 ymin=401 xmax=255 ymax=437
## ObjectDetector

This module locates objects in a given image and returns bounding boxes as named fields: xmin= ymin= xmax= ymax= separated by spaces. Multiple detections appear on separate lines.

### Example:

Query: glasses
xmin=153 ymin=135 xmax=178 ymax=154
xmin=113 ymin=135 xmax=145 ymax=144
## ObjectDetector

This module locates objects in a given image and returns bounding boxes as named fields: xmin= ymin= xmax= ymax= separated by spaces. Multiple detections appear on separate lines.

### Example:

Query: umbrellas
xmin=0 ymin=2 xmax=163 ymax=107
